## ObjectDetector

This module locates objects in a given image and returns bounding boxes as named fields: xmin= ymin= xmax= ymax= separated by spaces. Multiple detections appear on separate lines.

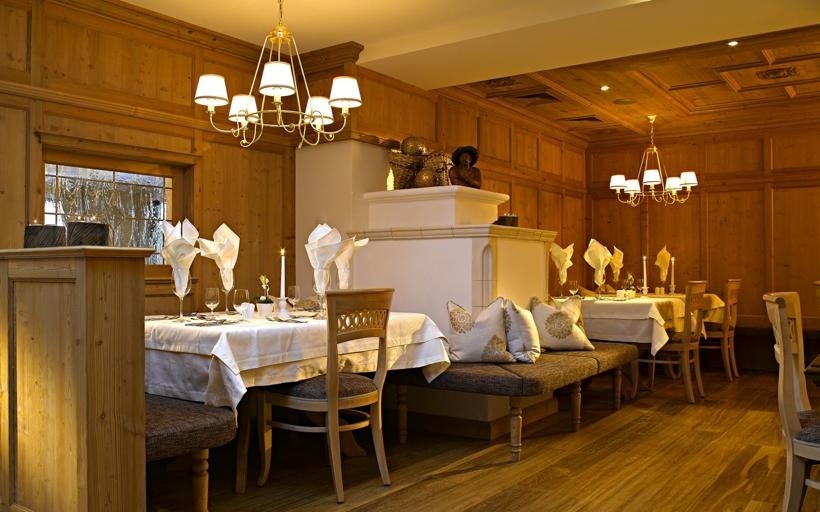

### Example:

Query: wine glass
xmin=232 ymin=288 xmax=249 ymax=316
xmin=594 ymin=270 xmax=606 ymax=300
xmin=567 ymin=280 xmax=579 ymax=297
xmin=287 ymin=286 xmax=301 ymax=311
xmin=311 ymin=268 xmax=331 ymax=320
xmin=204 ymin=288 xmax=219 ymax=318
xmin=612 ymin=269 xmax=620 ymax=291
xmin=219 ymin=269 xmax=235 ymax=313
xmin=170 ymin=271 xmax=191 ymax=323
xmin=335 ymin=267 xmax=353 ymax=291
xmin=556 ymin=270 xmax=567 ymax=299
xmin=660 ymin=269 xmax=668 ymax=295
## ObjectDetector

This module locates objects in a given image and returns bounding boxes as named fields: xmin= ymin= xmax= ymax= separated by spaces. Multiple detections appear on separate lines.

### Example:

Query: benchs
xmin=386 ymin=351 xmax=598 ymax=464
xmin=543 ymin=316 xmax=640 ymax=412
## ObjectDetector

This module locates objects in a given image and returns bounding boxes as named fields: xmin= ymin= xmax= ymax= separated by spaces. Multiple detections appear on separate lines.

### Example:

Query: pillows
xmin=530 ymin=295 xmax=595 ymax=352
xmin=447 ymin=297 xmax=518 ymax=363
xmin=548 ymin=295 xmax=585 ymax=336
xmin=504 ymin=298 xmax=541 ymax=363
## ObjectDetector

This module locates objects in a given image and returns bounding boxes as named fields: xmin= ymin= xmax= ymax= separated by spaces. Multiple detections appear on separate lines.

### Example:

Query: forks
xmin=273 ymin=316 xmax=306 ymax=323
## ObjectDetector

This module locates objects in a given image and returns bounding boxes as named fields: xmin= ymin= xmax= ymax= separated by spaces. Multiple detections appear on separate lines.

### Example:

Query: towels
xmin=334 ymin=237 xmax=369 ymax=290
xmin=584 ymin=238 xmax=611 ymax=284
xmin=609 ymin=245 xmax=624 ymax=281
xmin=304 ymin=222 xmax=356 ymax=293
xmin=551 ymin=242 xmax=574 ymax=283
xmin=196 ymin=222 xmax=240 ymax=290
xmin=655 ymin=244 xmax=671 ymax=282
xmin=157 ymin=217 xmax=200 ymax=298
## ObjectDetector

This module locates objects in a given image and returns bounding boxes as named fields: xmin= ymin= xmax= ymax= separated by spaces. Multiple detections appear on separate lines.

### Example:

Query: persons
xmin=450 ymin=145 xmax=483 ymax=188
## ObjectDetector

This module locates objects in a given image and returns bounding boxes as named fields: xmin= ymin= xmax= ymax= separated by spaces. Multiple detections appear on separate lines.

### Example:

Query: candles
xmin=280 ymin=248 xmax=285 ymax=314
xmin=671 ymin=256 xmax=675 ymax=285
xmin=643 ymin=255 xmax=647 ymax=287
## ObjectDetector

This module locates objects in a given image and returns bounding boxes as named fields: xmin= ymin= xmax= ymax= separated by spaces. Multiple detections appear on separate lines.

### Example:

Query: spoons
xmin=265 ymin=315 xmax=281 ymax=321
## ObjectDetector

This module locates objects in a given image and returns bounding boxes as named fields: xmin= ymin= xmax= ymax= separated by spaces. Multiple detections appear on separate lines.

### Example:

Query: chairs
xmin=144 ymin=392 xmax=236 ymax=512
xmin=608 ymin=115 xmax=699 ymax=208
xmin=803 ymin=280 xmax=820 ymax=388
xmin=631 ymin=280 xmax=707 ymax=403
xmin=257 ymin=286 xmax=394 ymax=503
xmin=763 ymin=290 xmax=820 ymax=512
xmin=677 ymin=279 xmax=741 ymax=382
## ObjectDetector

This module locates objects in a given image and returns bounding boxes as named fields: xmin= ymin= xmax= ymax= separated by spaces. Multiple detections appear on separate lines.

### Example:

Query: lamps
xmin=192 ymin=1 xmax=361 ymax=148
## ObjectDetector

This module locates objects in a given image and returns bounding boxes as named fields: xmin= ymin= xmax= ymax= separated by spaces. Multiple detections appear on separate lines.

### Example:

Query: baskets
xmin=385 ymin=151 xmax=423 ymax=190
xmin=423 ymin=150 xmax=448 ymax=186
